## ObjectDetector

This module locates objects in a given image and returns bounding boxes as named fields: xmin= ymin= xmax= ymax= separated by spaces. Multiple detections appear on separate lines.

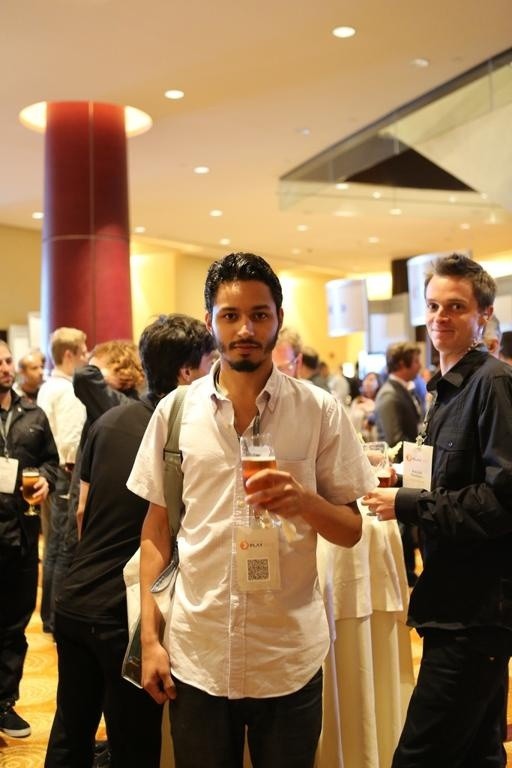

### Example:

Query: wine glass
xmin=22 ymin=467 xmax=40 ymax=516
xmin=240 ymin=431 xmax=287 ymax=530
xmin=364 ymin=440 xmax=397 ymax=518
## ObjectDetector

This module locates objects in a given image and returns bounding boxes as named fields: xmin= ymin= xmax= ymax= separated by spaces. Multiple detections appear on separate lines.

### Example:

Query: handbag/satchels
xmin=121 ymin=538 xmax=180 ymax=689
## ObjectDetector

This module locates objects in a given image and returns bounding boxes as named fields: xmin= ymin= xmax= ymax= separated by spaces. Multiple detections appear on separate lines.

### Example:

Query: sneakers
xmin=0 ymin=701 xmax=31 ymax=737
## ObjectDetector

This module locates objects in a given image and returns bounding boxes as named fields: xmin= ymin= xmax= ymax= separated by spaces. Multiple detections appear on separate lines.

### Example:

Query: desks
xmin=306 ymin=494 xmax=417 ymax=767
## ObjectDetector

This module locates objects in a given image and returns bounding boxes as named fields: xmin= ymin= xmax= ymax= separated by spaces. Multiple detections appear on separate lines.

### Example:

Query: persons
xmin=63 ymin=335 xmax=146 ymax=573
xmin=13 ymin=349 xmax=47 ymax=407
xmin=271 ymin=325 xmax=512 ymax=443
xmin=0 ymin=322 xmax=62 ymax=740
xmin=375 ymin=340 xmax=427 ymax=588
xmin=357 ymin=246 xmax=511 ymax=767
xmin=123 ymin=249 xmax=384 ymax=768
xmin=35 ymin=325 xmax=93 ymax=640
xmin=42 ymin=311 xmax=218 ymax=767
